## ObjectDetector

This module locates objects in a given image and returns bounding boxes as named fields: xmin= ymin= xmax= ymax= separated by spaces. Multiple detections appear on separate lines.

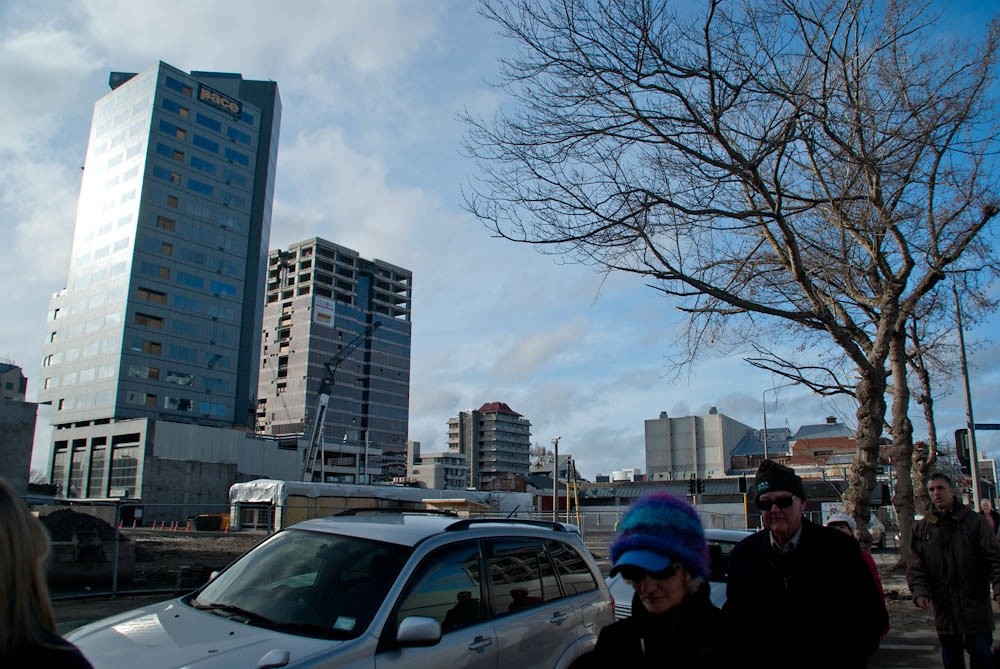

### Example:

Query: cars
xmin=604 ymin=528 xmax=760 ymax=626
xmin=868 ymin=512 xmax=887 ymax=549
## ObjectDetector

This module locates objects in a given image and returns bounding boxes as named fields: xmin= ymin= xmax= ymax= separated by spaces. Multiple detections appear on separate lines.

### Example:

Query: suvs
xmin=58 ymin=509 xmax=620 ymax=669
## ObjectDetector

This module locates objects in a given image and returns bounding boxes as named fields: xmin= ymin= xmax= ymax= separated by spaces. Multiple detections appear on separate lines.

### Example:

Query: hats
xmin=754 ymin=460 xmax=806 ymax=505
xmin=826 ymin=513 xmax=856 ymax=533
xmin=610 ymin=493 xmax=709 ymax=579
xmin=509 ymin=585 xmax=528 ymax=596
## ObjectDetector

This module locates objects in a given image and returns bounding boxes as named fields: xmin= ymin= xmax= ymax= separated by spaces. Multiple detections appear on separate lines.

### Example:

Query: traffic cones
xmin=185 ymin=521 xmax=191 ymax=531
xmin=224 ymin=523 xmax=229 ymax=533
xmin=119 ymin=518 xmax=123 ymax=529
xmin=152 ymin=518 xmax=179 ymax=531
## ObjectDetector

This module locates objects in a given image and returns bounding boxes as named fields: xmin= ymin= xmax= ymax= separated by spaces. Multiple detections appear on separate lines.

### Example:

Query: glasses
xmin=626 ymin=562 xmax=685 ymax=583
xmin=756 ymin=494 xmax=797 ymax=510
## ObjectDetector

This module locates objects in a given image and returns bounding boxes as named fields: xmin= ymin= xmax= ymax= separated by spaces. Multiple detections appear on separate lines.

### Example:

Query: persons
xmin=724 ymin=458 xmax=1000 ymax=669
xmin=441 ymin=585 xmax=544 ymax=631
xmin=0 ymin=479 xmax=97 ymax=669
xmin=588 ymin=494 xmax=803 ymax=669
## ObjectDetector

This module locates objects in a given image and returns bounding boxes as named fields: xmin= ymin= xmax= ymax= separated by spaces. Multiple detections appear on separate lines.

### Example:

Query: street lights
xmin=762 ymin=381 xmax=802 ymax=461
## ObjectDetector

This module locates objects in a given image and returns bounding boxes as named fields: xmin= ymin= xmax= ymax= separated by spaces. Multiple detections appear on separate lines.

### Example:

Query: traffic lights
xmin=739 ymin=475 xmax=747 ymax=495
xmin=697 ymin=478 xmax=705 ymax=493
xmin=688 ymin=480 xmax=695 ymax=493
